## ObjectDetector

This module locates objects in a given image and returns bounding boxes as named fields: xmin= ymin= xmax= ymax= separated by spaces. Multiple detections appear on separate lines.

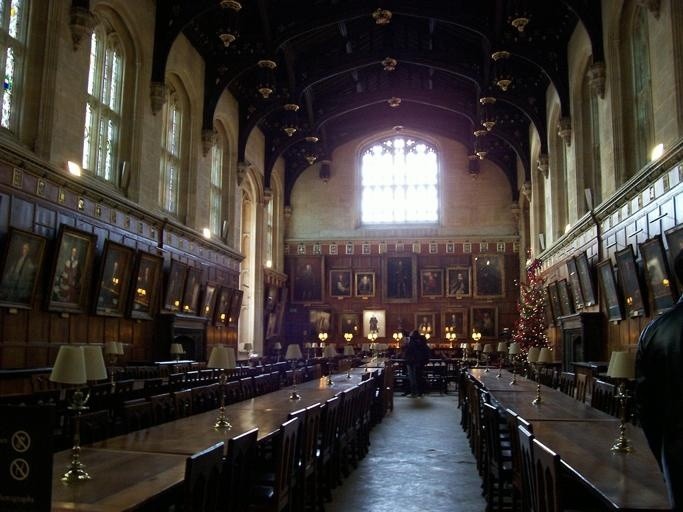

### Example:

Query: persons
xmin=635 ymin=248 xmax=683 ymax=512
xmin=293 ymin=264 xmax=316 ymax=301
xmin=419 ymin=315 xmax=431 ymax=331
xmin=2 ymin=242 xmax=154 ymax=313
xmin=359 ymin=276 xmax=371 ymax=295
xmin=447 ymin=313 xmax=461 ymax=333
xmin=333 ymin=272 xmax=350 ymax=295
xmin=474 ymin=311 xmax=493 ymax=334
xmin=401 ymin=330 xmax=429 ymax=398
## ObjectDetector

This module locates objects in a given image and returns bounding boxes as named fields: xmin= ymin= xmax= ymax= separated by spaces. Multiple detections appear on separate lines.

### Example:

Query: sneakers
xmin=407 ymin=392 xmax=425 ymax=399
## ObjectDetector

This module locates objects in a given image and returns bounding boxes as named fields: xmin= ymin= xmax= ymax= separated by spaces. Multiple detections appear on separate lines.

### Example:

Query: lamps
xmin=169 ymin=342 xmax=369 ymax=428
xmin=48 ymin=341 xmax=126 ymax=482
xmin=473 ymin=341 xmax=552 ymax=406
xmin=606 ymin=350 xmax=636 ymax=452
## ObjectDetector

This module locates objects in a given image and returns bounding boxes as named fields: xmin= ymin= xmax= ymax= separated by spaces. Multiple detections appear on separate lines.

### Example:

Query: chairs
xmin=458 ymin=361 xmax=678 ymax=511
xmin=1 ymin=351 xmax=400 ymax=512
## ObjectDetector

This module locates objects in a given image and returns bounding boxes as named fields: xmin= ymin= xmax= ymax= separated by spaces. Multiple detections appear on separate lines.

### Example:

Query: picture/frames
xmin=544 ymin=225 xmax=683 ymax=330
xmin=0 ymin=222 xmax=244 ymax=331
xmin=288 ymin=254 xmax=507 ymax=341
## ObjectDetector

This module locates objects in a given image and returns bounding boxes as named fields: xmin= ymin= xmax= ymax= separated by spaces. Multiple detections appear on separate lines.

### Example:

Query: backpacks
xmin=415 ymin=344 xmax=432 ymax=368
